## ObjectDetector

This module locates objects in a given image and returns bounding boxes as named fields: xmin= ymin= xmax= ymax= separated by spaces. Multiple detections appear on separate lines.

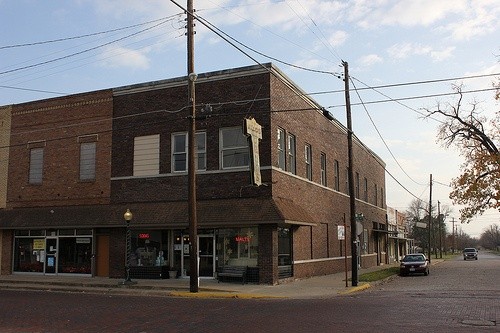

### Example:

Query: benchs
xmin=217 ymin=264 xmax=248 ymax=285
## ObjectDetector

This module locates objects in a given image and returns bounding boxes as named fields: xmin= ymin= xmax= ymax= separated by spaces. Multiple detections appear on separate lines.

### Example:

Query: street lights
xmin=122 ymin=208 xmax=137 ymax=286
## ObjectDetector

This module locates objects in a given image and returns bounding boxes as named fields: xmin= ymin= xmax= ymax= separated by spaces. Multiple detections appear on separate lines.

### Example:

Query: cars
xmin=399 ymin=253 xmax=430 ymax=276
xmin=463 ymin=247 xmax=477 ymax=260
